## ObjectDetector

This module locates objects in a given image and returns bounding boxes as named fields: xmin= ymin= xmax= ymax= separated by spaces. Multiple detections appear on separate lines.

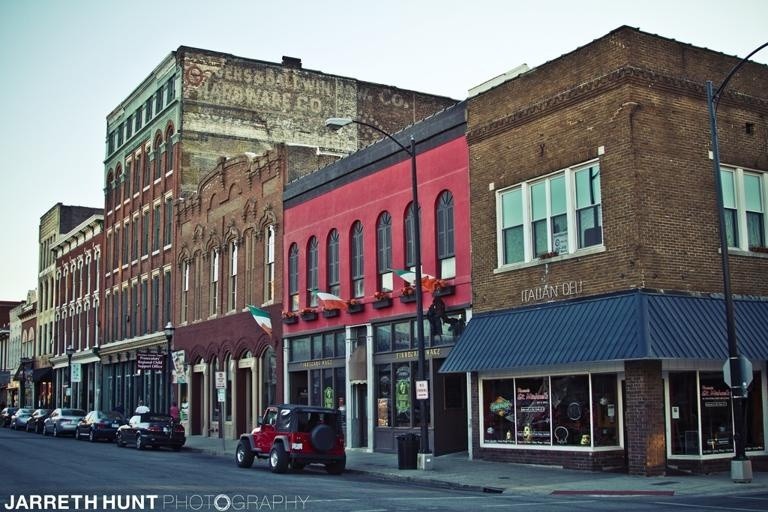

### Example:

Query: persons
xmin=170 ymin=402 xmax=180 ymax=422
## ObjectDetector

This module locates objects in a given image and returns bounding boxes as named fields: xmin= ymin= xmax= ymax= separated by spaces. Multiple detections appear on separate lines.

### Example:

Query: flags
xmin=248 ymin=305 xmax=273 ymax=338
xmin=310 ymin=290 xmax=349 ymax=310
xmin=394 ymin=269 xmax=438 ymax=293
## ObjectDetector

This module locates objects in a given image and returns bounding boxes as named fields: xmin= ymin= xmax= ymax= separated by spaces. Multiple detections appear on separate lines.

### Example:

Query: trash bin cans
xmin=392 ymin=433 xmax=423 ymax=470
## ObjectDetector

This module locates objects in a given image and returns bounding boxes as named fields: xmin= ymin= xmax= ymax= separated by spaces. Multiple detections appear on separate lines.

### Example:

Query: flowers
xmin=422 ymin=279 xmax=453 ymax=292
xmin=281 ymin=309 xmax=297 ymax=317
xmin=365 ymin=291 xmax=394 ymax=301
xmin=315 ymin=298 xmax=345 ymax=311
xmin=298 ymin=306 xmax=317 ymax=314
xmin=342 ymin=297 xmax=368 ymax=306
xmin=397 ymin=286 xmax=417 ymax=297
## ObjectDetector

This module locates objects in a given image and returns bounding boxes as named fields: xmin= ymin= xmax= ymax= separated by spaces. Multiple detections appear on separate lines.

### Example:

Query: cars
xmin=0 ymin=407 xmax=19 ymax=427
xmin=26 ymin=409 xmax=54 ymax=434
xmin=10 ymin=408 xmax=35 ymax=431
xmin=42 ymin=407 xmax=87 ymax=436
xmin=116 ymin=412 xmax=186 ymax=452
xmin=75 ymin=409 xmax=129 ymax=443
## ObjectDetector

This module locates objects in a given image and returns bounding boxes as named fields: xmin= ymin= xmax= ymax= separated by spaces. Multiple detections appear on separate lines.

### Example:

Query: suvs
xmin=236 ymin=405 xmax=347 ymax=475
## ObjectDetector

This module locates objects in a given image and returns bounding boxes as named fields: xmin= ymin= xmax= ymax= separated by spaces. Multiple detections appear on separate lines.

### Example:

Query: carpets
xmin=547 ymin=489 xmax=675 ymax=498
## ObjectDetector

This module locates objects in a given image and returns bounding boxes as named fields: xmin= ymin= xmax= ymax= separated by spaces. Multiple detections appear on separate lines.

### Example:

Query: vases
xmin=399 ymin=293 xmax=418 ymax=303
xmin=370 ymin=298 xmax=394 ymax=309
xmin=433 ymin=284 xmax=456 ymax=298
xmin=301 ymin=311 xmax=318 ymax=322
xmin=347 ymin=303 xmax=365 ymax=314
xmin=282 ymin=316 xmax=298 ymax=324
xmin=322 ymin=308 xmax=339 ymax=320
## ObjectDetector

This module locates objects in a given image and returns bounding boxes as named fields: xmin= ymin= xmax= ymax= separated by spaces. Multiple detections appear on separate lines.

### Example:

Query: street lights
xmin=66 ymin=344 xmax=75 ymax=408
xmin=324 ymin=116 xmax=435 ymax=471
xmin=164 ymin=319 xmax=175 ymax=414
